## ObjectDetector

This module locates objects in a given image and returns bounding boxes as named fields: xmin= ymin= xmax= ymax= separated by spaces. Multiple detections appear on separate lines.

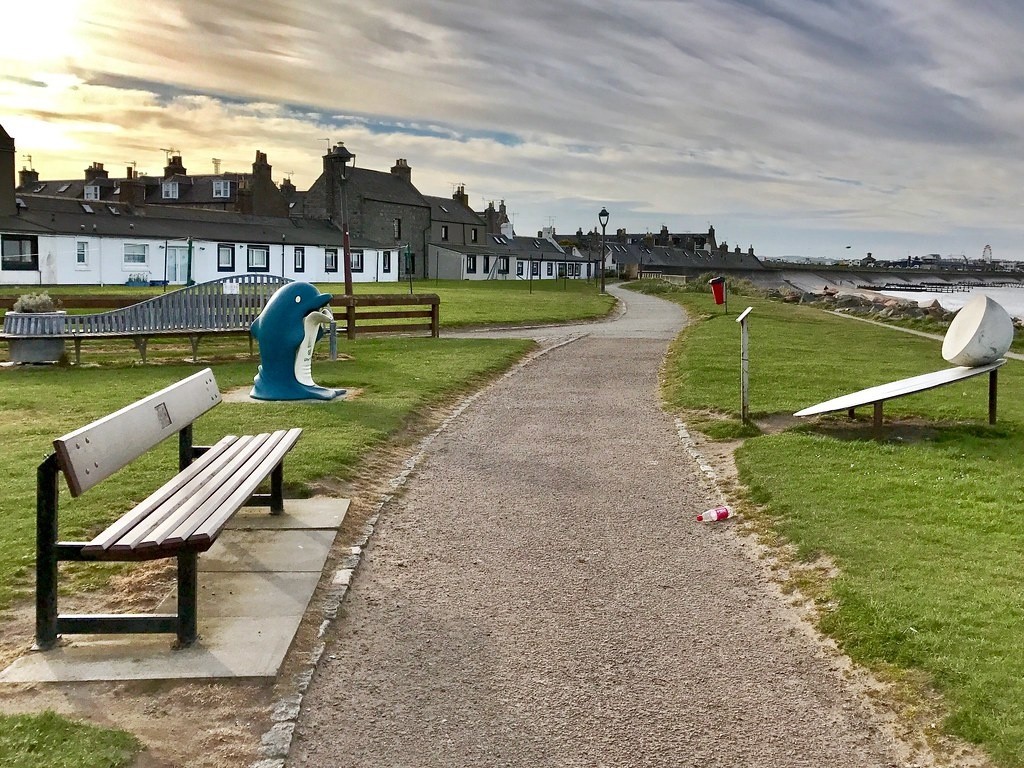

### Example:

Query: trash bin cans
xmin=249 ymin=280 xmax=347 ymax=401
xmin=709 ymin=277 xmax=725 ymax=305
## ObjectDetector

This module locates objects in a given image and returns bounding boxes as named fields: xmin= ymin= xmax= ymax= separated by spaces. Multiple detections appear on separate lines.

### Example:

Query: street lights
xmin=325 ymin=140 xmax=357 ymax=339
xmin=598 ymin=206 xmax=610 ymax=294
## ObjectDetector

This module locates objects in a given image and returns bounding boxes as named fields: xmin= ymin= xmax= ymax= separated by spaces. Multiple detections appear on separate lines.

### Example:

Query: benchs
xmin=36 ymin=368 xmax=302 ymax=649
